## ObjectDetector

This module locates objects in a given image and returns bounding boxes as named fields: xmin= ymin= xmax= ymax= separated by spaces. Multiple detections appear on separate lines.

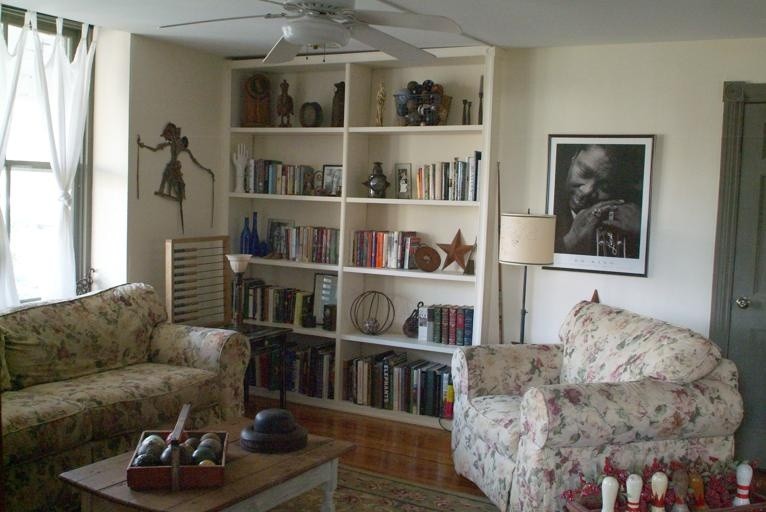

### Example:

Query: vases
xmin=250 ymin=212 xmax=259 ymax=256
xmin=241 ymin=217 xmax=252 ymax=254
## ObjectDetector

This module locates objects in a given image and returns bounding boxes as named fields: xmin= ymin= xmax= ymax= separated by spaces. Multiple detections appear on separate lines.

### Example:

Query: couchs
xmin=0 ymin=283 xmax=252 ymax=512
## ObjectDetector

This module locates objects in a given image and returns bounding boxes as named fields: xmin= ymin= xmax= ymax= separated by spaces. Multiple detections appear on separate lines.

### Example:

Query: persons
xmin=553 ymin=142 xmax=642 ymax=260
xmin=272 ymin=225 xmax=287 ymax=255
xmin=325 ymin=169 xmax=342 ymax=195
xmin=232 ymin=144 xmax=248 ymax=192
xmin=136 ymin=121 xmax=215 ymax=202
xmin=399 ymin=170 xmax=408 ymax=193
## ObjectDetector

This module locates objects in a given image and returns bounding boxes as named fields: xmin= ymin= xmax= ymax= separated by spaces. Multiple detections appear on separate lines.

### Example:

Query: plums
xmin=395 ymin=79 xmax=449 ymax=126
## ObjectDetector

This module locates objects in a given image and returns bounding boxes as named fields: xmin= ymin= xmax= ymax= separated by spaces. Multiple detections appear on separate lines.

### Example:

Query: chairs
xmin=451 ymin=301 xmax=744 ymax=512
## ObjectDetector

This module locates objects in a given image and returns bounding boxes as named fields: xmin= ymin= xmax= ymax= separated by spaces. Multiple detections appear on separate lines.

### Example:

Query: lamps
xmin=282 ymin=15 xmax=350 ymax=62
xmin=226 ymin=254 xmax=253 ymax=328
xmin=498 ymin=209 xmax=557 ymax=343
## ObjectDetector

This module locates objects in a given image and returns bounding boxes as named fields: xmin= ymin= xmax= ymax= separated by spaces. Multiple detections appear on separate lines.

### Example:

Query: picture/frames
xmin=395 ymin=163 xmax=412 ymax=199
xmin=322 ymin=165 xmax=342 ymax=196
xmin=542 ymin=134 xmax=655 ymax=277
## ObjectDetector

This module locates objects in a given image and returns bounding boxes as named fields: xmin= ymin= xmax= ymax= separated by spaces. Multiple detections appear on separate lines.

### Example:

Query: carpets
xmin=267 ymin=462 xmax=499 ymax=512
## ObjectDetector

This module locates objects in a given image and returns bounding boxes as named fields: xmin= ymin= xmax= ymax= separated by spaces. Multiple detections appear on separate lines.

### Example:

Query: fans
xmin=161 ymin=0 xmax=464 ymax=65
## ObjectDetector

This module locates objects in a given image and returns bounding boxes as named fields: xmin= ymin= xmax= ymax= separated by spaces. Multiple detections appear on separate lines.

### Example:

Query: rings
xmin=591 ymin=207 xmax=602 ymax=218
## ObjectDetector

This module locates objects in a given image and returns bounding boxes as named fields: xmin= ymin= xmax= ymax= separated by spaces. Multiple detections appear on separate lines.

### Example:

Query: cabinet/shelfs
xmin=218 ymin=46 xmax=530 ymax=430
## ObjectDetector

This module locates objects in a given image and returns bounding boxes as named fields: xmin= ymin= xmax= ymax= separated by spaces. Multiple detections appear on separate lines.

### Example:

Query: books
xmin=244 ymin=158 xmax=314 ymax=195
xmin=242 ymin=329 xmax=335 ymax=401
xmin=284 ymin=226 xmax=340 ymax=266
xmin=349 ymin=228 xmax=421 ymax=270
xmin=416 ymin=150 xmax=480 ymax=202
xmin=341 ymin=350 xmax=453 ymax=420
xmin=418 ymin=304 xmax=474 ymax=346
xmin=232 ymin=278 xmax=313 ymax=327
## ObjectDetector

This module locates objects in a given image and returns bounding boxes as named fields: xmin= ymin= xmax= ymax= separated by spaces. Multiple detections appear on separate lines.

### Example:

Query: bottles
xmin=241 ymin=212 xmax=258 ymax=256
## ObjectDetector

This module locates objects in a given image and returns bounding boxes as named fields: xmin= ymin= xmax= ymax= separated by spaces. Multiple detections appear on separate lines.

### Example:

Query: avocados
xmin=131 ymin=433 xmax=223 ymax=467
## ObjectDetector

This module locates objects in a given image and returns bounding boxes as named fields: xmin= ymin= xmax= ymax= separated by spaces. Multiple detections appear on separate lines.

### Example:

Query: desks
xmin=207 ymin=323 xmax=293 ymax=417
xmin=57 ymin=417 xmax=358 ymax=512
